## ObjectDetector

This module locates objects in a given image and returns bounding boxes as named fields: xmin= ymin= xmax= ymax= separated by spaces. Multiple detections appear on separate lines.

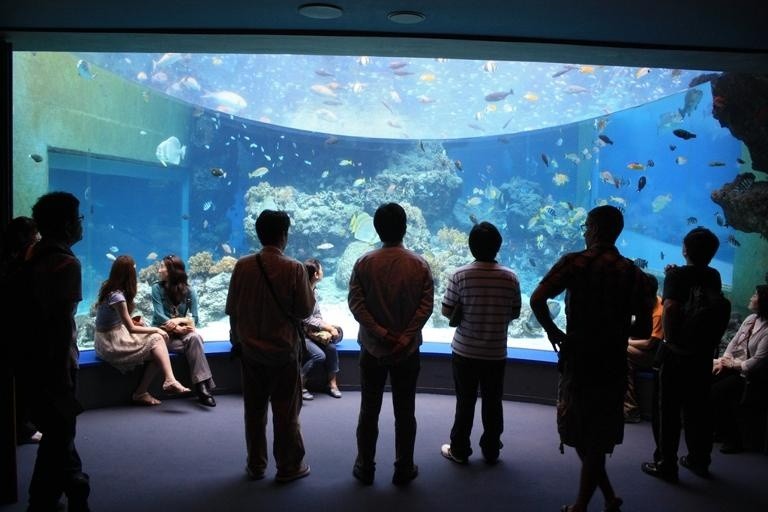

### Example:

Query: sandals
xmin=133 ymin=391 xmax=162 ymax=405
xmin=162 ymin=378 xmax=192 ymax=393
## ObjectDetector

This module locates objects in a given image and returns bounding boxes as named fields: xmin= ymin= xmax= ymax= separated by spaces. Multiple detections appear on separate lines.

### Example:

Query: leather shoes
xmin=197 ymin=381 xmax=211 ymax=398
xmin=198 ymin=384 xmax=216 ymax=406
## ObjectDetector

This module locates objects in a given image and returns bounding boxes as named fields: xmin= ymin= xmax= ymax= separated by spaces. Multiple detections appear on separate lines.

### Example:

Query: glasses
xmin=168 ymin=255 xmax=174 ymax=264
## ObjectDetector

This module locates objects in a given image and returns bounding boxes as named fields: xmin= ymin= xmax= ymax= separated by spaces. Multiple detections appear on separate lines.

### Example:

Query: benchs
xmin=77 ymin=339 xmax=657 ymax=421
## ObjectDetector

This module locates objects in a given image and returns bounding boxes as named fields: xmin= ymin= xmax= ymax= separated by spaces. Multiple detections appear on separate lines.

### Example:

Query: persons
xmin=225 ymin=208 xmax=310 ymax=484
xmin=529 ymin=204 xmax=653 ymax=511
xmin=10 ymin=216 xmax=43 ymax=446
xmin=347 ymin=203 xmax=433 ymax=486
xmin=94 ymin=255 xmax=191 ymax=405
xmin=624 ymin=274 xmax=664 ymax=425
xmin=301 ymin=259 xmax=343 ymax=401
xmin=642 ymin=228 xmax=730 ymax=484
xmin=442 ymin=221 xmax=521 ymax=466
xmin=23 ymin=191 xmax=90 ymax=512
xmin=713 ymin=285 xmax=767 ymax=454
xmin=151 ymin=256 xmax=217 ymax=407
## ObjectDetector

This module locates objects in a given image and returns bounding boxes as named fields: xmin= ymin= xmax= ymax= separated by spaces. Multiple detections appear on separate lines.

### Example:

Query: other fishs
xmin=13 ymin=53 xmax=768 ymax=298
xmin=525 ymin=298 xmax=561 ymax=331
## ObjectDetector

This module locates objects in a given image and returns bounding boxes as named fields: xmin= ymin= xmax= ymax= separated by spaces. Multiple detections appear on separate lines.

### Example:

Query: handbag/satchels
xmin=307 ymin=326 xmax=343 ymax=346
